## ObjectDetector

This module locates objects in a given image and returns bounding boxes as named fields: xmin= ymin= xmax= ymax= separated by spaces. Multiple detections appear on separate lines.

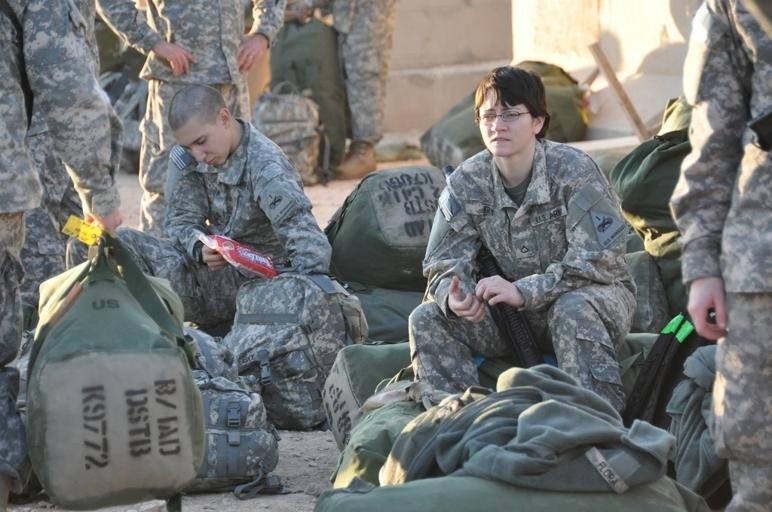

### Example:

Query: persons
xmin=669 ymin=0 xmax=772 ymax=511
xmin=1 ymin=0 xmax=391 ymax=502
xmin=409 ymin=67 xmax=636 ymax=414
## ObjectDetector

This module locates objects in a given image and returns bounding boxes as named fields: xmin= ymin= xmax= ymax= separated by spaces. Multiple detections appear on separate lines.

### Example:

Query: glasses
xmin=477 ymin=111 xmax=531 ymax=121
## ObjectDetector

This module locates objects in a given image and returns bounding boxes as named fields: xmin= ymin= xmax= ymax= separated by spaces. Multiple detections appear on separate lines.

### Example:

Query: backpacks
xmin=254 ymin=80 xmax=333 ymax=185
xmin=184 ymin=326 xmax=282 ymax=496
xmin=26 ymin=229 xmax=202 ymax=512
xmin=228 ymin=273 xmax=351 ymax=430
xmin=19 ymin=205 xmax=67 ymax=312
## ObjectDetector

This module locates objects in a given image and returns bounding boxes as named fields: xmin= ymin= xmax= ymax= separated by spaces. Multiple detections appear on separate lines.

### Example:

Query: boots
xmin=336 ymin=142 xmax=376 ymax=179
